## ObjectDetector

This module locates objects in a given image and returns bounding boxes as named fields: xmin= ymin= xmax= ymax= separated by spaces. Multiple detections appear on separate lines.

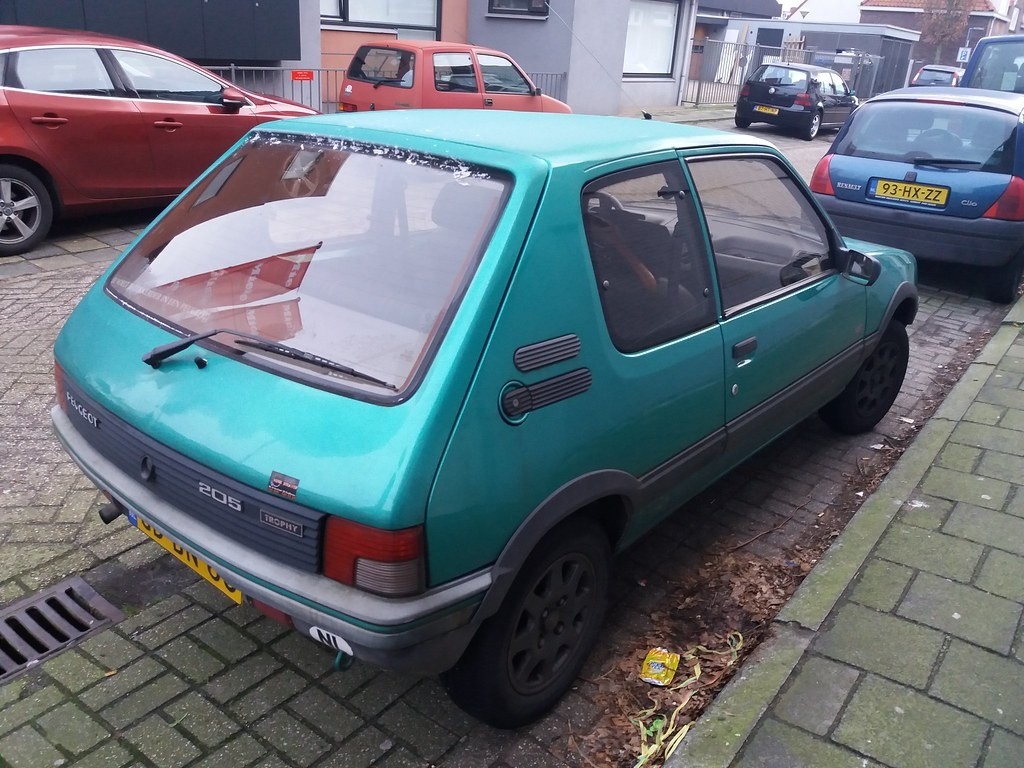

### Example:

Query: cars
xmin=910 ymin=64 xmax=968 ymax=89
xmin=0 ymin=22 xmax=343 ymax=257
xmin=800 ymin=86 xmax=1024 ymax=303
xmin=48 ymin=107 xmax=920 ymax=730
xmin=735 ymin=62 xmax=861 ymax=142
xmin=337 ymin=39 xmax=572 ymax=114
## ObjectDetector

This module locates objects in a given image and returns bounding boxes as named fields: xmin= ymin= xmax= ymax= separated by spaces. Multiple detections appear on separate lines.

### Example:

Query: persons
xmin=587 ymin=211 xmax=657 ymax=292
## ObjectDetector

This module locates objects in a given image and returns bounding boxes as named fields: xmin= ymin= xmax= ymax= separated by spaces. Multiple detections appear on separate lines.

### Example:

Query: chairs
xmin=448 ymin=65 xmax=477 ymax=93
xmin=620 ymin=218 xmax=702 ymax=337
xmin=898 ymin=108 xmax=934 ymax=146
xmin=430 ymin=183 xmax=500 ymax=229
xmin=765 ymin=73 xmax=806 ymax=87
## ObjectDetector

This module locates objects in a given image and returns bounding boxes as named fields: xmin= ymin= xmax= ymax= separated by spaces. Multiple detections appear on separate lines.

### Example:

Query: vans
xmin=959 ymin=33 xmax=1024 ymax=96
